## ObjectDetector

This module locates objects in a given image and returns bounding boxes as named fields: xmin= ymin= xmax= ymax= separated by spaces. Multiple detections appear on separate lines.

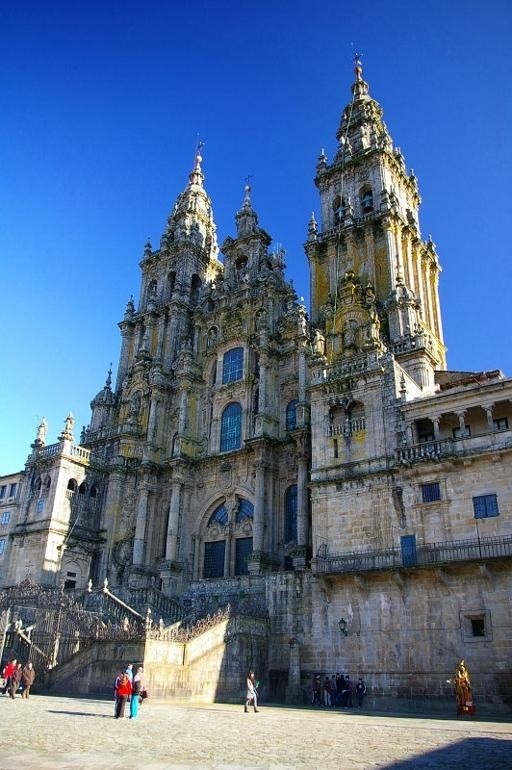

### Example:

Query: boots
xmin=254 ymin=707 xmax=258 ymax=712
xmin=245 ymin=707 xmax=248 ymax=712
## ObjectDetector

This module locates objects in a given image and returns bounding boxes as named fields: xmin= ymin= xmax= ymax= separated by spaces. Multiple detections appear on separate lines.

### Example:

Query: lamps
xmin=338 ymin=618 xmax=349 ymax=636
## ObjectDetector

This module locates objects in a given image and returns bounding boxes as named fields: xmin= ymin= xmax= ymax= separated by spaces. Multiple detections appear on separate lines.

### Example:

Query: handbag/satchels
xmin=140 ymin=690 xmax=146 ymax=697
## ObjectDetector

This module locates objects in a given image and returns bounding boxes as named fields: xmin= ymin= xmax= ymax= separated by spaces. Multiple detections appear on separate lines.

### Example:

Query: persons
xmin=312 ymin=672 xmax=353 ymax=710
xmin=244 ymin=669 xmax=260 ymax=713
xmin=355 ymin=677 xmax=367 ymax=710
xmin=446 ymin=659 xmax=475 ymax=716
xmin=112 ymin=664 xmax=143 ymax=719
xmin=2 ymin=657 xmax=36 ymax=700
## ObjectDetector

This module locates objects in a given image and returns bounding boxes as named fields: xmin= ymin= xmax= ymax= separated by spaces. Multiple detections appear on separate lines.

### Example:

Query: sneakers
xmin=113 ymin=715 xmax=133 ymax=720
